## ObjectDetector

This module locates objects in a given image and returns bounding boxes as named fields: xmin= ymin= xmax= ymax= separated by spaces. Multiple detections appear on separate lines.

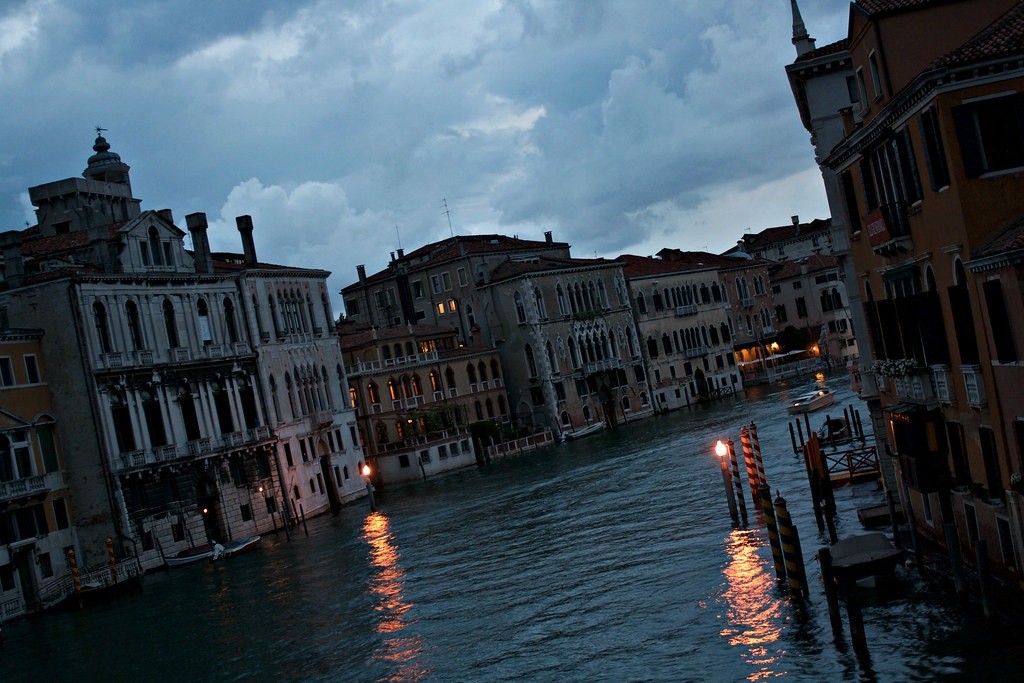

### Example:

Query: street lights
xmin=715 ymin=439 xmax=739 ymax=523
xmin=362 ymin=463 xmax=376 ymax=510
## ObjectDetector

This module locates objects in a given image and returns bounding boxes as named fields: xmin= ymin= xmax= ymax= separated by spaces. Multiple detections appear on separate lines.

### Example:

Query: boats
xmin=819 ymin=415 xmax=847 ymax=441
xmin=786 ymin=385 xmax=838 ymax=415
xmin=163 ymin=545 xmax=215 ymax=567
xmin=221 ymin=533 xmax=261 ymax=558
